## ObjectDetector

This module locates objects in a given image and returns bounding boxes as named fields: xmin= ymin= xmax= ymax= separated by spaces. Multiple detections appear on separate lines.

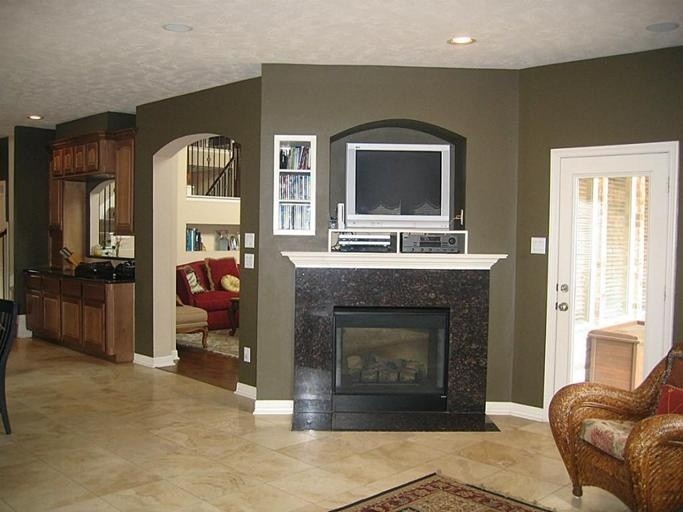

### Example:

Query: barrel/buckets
xmin=218 ymin=230 xmax=228 ymax=251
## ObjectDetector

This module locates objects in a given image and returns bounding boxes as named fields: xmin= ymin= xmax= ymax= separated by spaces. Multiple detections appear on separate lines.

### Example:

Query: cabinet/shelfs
xmin=273 ymin=134 xmax=316 ymax=236
xmin=24 ymin=269 xmax=134 ymax=363
xmin=113 ymin=128 xmax=136 ymax=235
xmin=48 ymin=155 xmax=63 ymax=231
xmin=48 ymin=131 xmax=115 ymax=181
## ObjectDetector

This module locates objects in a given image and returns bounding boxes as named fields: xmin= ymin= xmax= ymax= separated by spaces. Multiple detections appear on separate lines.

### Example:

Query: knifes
xmin=59 ymin=247 xmax=73 ymax=257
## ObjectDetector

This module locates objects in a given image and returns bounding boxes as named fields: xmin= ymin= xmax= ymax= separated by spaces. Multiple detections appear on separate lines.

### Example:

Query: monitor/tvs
xmin=346 ymin=142 xmax=455 ymax=232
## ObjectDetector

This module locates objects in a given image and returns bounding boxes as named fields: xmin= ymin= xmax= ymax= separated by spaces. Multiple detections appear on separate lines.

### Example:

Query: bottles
xmin=186 ymin=226 xmax=201 ymax=251
xmin=215 ymin=230 xmax=237 ymax=251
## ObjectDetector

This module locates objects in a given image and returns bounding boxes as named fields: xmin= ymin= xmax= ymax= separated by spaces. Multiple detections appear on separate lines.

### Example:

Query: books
xmin=184 ymin=226 xmax=202 ymax=252
xmin=277 ymin=144 xmax=311 ymax=230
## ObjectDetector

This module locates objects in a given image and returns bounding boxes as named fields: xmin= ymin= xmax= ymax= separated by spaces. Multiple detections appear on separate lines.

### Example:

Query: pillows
xmin=205 ymin=257 xmax=240 ymax=292
xmin=176 ymin=294 xmax=184 ymax=306
xmin=185 ymin=270 xmax=205 ymax=294
xmin=221 ymin=274 xmax=240 ymax=292
xmin=651 ymin=350 xmax=683 ymax=415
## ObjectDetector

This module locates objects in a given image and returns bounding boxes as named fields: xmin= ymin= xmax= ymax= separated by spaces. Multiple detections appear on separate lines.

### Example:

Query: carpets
xmin=176 ymin=329 xmax=240 ymax=358
xmin=327 ymin=473 xmax=555 ymax=512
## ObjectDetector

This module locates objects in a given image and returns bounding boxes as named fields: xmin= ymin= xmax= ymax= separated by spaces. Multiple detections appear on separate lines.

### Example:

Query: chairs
xmin=176 ymin=294 xmax=209 ymax=348
xmin=549 ymin=344 xmax=683 ymax=512
xmin=0 ymin=299 xmax=17 ymax=435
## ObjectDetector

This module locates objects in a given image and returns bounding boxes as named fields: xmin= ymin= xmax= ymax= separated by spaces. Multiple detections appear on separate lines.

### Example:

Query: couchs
xmin=176 ymin=261 xmax=240 ymax=330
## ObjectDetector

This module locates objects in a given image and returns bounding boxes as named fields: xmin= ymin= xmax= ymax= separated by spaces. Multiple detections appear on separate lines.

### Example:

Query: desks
xmin=585 ymin=320 xmax=646 ymax=393
